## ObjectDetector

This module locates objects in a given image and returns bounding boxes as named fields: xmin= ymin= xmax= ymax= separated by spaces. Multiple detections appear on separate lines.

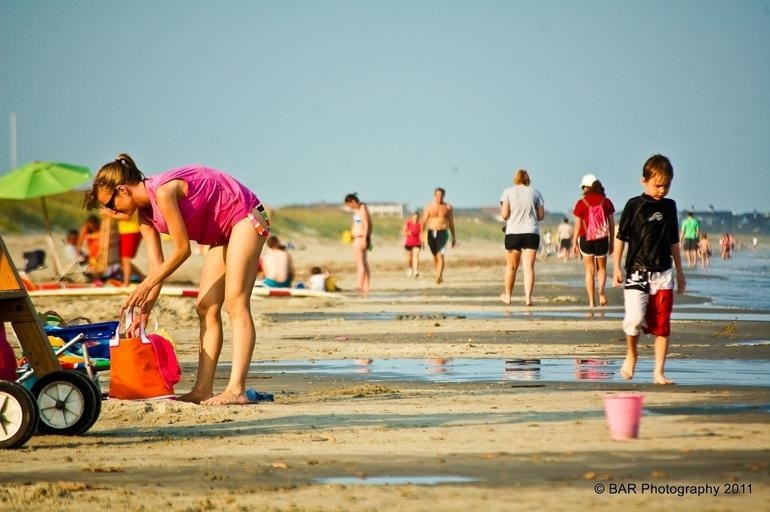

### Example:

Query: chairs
xmin=46 ymin=236 xmax=86 ymax=283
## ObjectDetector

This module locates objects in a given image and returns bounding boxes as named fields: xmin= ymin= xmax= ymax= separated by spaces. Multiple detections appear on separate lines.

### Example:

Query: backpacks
xmin=581 ymin=197 xmax=610 ymax=241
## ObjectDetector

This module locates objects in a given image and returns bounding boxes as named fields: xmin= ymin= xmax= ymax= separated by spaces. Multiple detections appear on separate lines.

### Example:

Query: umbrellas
xmin=0 ymin=160 xmax=92 ymax=276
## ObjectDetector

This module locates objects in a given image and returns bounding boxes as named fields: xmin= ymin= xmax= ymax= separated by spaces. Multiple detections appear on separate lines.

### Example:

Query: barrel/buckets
xmin=600 ymin=394 xmax=646 ymax=441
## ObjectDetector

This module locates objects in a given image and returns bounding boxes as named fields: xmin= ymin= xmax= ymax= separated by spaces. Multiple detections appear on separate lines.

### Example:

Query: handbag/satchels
xmin=18 ymin=311 xmax=117 ymax=371
xmin=109 ymin=304 xmax=181 ymax=399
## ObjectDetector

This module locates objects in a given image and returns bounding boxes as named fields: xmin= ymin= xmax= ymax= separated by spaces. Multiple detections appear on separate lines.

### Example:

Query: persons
xmin=305 ymin=266 xmax=330 ymax=291
xmin=402 ymin=212 xmax=425 ymax=278
xmin=680 ymin=210 xmax=734 ymax=270
xmin=63 ymin=229 xmax=147 ymax=281
xmin=421 ymin=187 xmax=457 ymax=284
xmin=100 ymin=207 xmax=143 ymax=288
xmin=536 ymin=214 xmax=585 ymax=266
xmin=344 ymin=191 xmax=374 ymax=293
xmin=85 ymin=214 xmax=102 ymax=249
xmin=263 ymin=236 xmax=295 ymax=288
xmin=84 ymin=153 xmax=269 ymax=406
xmin=611 ymin=152 xmax=687 ymax=387
xmin=571 ymin=174 xmax=618 ymax=308
xmin=752 ymin=234 xmax=759 ymax=248
xmin=499 ymin=169 xmax=546 ymax=305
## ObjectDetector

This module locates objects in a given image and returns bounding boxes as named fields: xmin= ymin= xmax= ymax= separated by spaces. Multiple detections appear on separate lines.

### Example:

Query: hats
xmin=581 ymin=174 xmax=597 ymax=188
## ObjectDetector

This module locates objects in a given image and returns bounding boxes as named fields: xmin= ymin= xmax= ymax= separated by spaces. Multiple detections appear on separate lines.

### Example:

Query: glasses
xmin=105 ymin=190 xmax=117 ymax=211
